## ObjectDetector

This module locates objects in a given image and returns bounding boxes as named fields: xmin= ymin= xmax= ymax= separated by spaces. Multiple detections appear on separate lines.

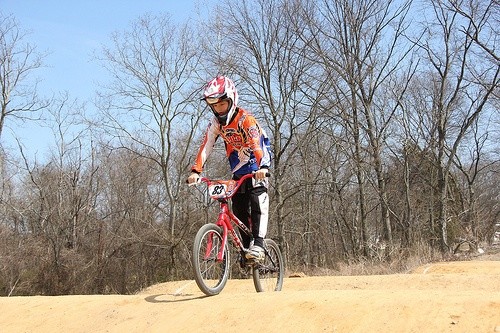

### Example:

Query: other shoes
xmin=245 ymin=245 xmax=265 ymax=264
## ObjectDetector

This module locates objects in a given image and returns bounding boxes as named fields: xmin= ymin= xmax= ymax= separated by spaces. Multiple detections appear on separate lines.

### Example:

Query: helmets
xmin=203 ymin=75 xmax=238 ymax=128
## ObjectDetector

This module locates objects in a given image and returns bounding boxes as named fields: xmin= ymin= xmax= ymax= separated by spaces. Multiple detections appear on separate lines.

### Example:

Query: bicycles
xmin=185 ymin=172 xmax=285 ymax=295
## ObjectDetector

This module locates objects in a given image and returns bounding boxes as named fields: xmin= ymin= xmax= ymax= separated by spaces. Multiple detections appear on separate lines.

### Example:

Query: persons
xmin=187 ymin=75 xmax=272 ymax=276
